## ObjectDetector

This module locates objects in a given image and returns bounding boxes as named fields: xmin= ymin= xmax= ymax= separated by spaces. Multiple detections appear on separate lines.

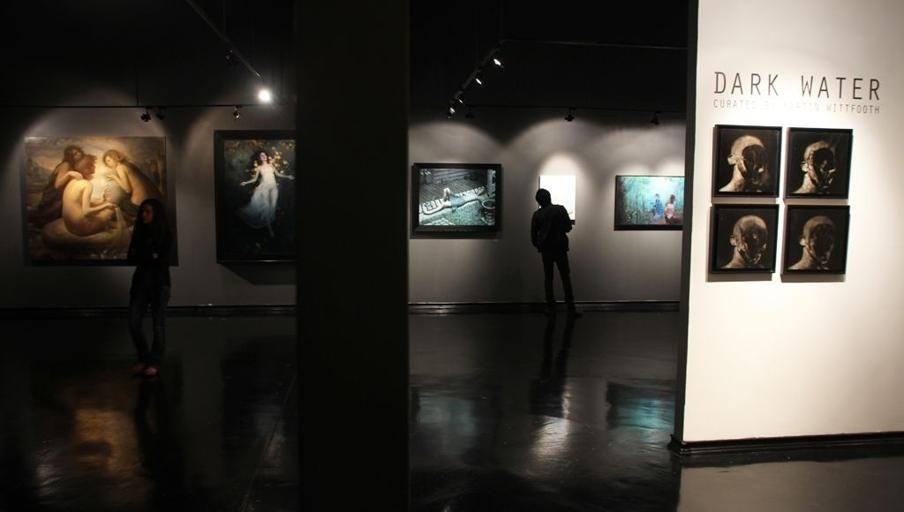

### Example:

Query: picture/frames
xmin=712 ymin=124 xmax=783 ymax=198
xmin=412 ymin=162 xmax=502 ymax=237
xmin=214 ymin=129 xmax=299 ymax=265
xmin=20 ymin=134 xmax=167 ymax=267
xmin=785 ymin=128 xmax=852 ymax=199
xmin=708 ymin=204 xmax=779 ymax=275
xmin=783 ymin=205 xmax=849 ymax=274
xmin=614 ymin=175 xmax=684 ymax=230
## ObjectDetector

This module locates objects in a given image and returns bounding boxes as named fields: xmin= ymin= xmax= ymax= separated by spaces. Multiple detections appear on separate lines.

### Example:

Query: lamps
xmin=141 ymin=108 xmax=151 ymax=123
xmin=650 ymin=111 xmax=662 ymax=126
xmin=232 ymin=109 xmax=240 ymax=119
xmin=447 ymin=46 xmax=505 ymax=119
xmin=465 ymin=107 xmax=476 ymax=120
xmin=155 ymin=108 xmax=167 ymax=121
xmin=564 ymin=107 xmax=576 ymax=122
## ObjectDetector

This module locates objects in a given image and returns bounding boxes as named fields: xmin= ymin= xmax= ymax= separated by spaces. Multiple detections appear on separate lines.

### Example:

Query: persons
xmin=722 ymin=214 xmax=768 ymax=269
xmin=664 ymin=195 xmax=680 ymax=223
xmin=791 ymin=140 xmax=838 ymax=193
xmin=39 ymin=145 xmax=84 ymax=214
xmin=531 ymin=189 xmax=583 ymax=319
xmin=240 ymin=151 xmax=296 ymax=238
xmin=103 ymin=149 xmax=162 ymax=218
xmin=718 ymin=135 xmax=769 ymax=192
xmin=126 ymin=198 xmax=178 ymax=375
xmin=654 ymin=194 xmax=663 ymax=215
xmin=62 ymin=154 xmax=120 ymax=236
xmin=423 ymin=184 xmax=488 ymax=215
xmin=789 ymin=216 xmax=837 ymax=270
